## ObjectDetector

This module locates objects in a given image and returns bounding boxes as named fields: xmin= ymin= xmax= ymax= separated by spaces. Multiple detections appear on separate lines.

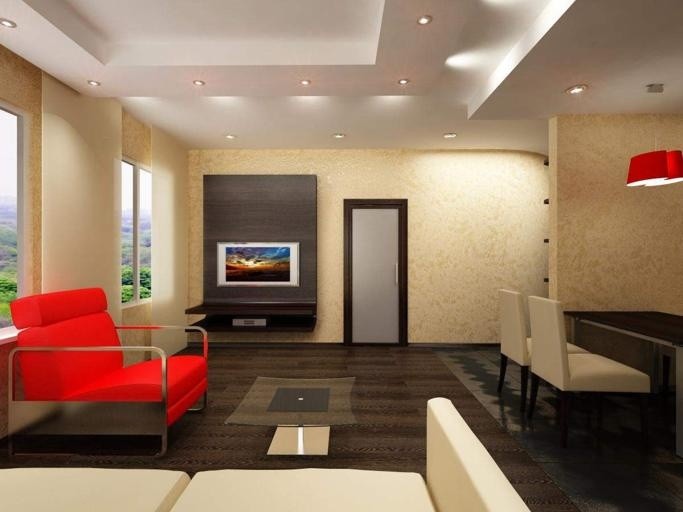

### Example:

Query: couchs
xmin=0 ymin=398 xmax=530 ymax=512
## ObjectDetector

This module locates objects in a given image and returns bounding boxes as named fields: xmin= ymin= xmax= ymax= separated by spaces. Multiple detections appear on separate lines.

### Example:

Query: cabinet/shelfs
xmin=184 ymin=303 xmax=317 ymax=332
xmin=543 ymin=159 xmax=549 ymax=283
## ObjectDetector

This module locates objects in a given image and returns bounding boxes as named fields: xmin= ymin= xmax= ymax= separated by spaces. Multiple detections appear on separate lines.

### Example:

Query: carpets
xmin=227 ymin=376 xmax=357 ymax=427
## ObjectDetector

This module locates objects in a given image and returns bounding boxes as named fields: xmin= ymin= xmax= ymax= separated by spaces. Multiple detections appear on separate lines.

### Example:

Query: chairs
xmin=497 ymin=288 xmax=588 ymax=413
xmin=8 ymin=289 xmax=209 ymax=460
xmin=529 ymin=295 xmax=651 ymax=456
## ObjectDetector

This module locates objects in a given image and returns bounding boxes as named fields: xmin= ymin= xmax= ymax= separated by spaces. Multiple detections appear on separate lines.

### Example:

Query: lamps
xmin=627 ymin=84 xmax=683 ymax=187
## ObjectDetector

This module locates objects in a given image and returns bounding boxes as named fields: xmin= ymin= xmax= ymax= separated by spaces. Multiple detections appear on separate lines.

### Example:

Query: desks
xmin=562 ymin=311 xmax=683 ymax=458
